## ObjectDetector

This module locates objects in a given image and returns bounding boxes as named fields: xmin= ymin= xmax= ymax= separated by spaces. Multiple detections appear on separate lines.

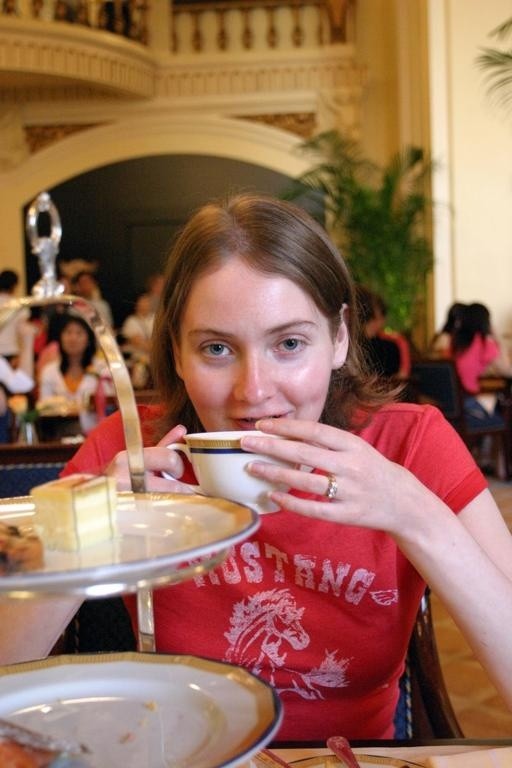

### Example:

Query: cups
xmin=156 ymin=428 xmax=305 ymax=517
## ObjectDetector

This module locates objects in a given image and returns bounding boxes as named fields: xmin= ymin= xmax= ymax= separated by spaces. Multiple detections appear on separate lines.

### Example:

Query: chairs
xmin=1 ymin=445 xmax=463 ymax=738
xmin=406 ymin=360 xmax=506 ymax=480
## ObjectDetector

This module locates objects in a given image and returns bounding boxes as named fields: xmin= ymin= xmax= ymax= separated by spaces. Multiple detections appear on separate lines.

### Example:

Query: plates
xmin=267 ymin=754 xmax=434 ymax=768
xmin=1 ymin=487 xmax=265 ymax=596
xmin=0 ymin=651 xmax=285 ymax=767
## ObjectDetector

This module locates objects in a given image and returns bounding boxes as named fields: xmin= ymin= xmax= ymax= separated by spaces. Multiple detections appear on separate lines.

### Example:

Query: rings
xmin=323 ymin=477 xmax=338 ymax=496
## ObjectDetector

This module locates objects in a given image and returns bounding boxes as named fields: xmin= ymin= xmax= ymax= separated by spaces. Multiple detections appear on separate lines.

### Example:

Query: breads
xmin=0 ymin=520 xmax=44 ymax=574
xmin=1 ymin=718 xmax=61 ymax=768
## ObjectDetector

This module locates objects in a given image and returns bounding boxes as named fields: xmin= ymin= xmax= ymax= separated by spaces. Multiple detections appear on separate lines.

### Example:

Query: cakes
xmin=32 ymin=473 xmax=119 ymax=550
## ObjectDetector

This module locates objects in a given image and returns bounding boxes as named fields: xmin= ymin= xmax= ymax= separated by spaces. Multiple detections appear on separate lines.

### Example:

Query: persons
xmin=1 ymin=270 xmax=165 ymax=442
xmin=362 ymin=292 xmax=512 ymax=476
xmin=0 ymin=192 xmax=512 ymax=741
